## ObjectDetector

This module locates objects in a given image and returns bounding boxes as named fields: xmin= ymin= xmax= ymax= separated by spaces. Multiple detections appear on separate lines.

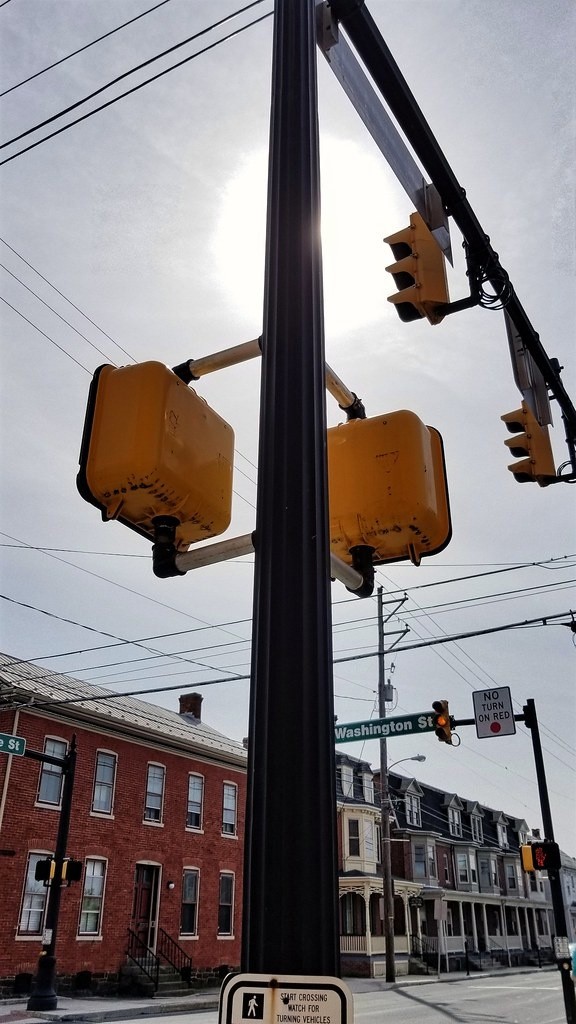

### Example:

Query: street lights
xmin=380 ymin=754 xmax=425 ymax=983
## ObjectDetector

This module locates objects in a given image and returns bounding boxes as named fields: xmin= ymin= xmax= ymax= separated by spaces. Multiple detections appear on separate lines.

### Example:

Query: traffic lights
xmin=501 ymin=400 xmax=555 ymax=488
xmin=35 ymin=860 xmax=56 ymax=881
xmin=519 ymin=846 xmax=536 ymax=873
xmin=530 ymin=840 xmax=561 ymax=871
xmin=431 ymin=699 xmax=452 ymax=742
xmin=383 ymin=211 xmax=450 ymax=325
xmin=62 ymin=860 xmax=82 ymax=882
